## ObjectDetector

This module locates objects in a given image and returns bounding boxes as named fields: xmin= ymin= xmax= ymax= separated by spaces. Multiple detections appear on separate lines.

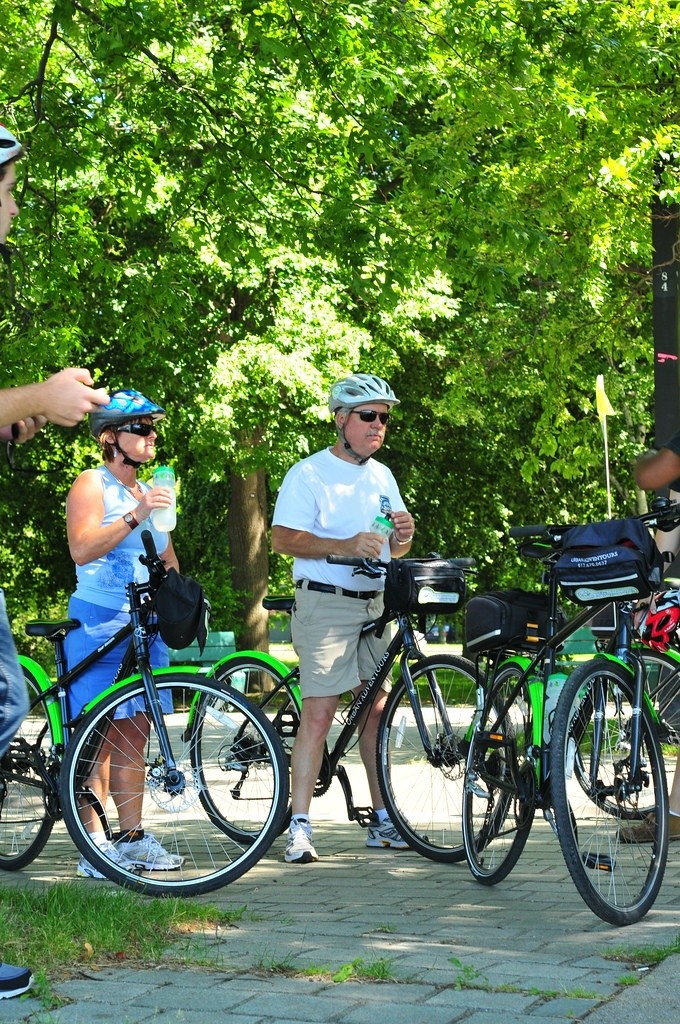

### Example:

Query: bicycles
xmin=0 ymin=528 xmax=290 ymax=898
xmin=180 ymin=551 xmax=519 ymax=862
xmin=460 ymin=497 xmax=680 ymax=925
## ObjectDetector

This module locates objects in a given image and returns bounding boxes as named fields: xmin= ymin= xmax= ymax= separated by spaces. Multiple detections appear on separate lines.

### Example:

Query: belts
xmin=296 ymin=579 xmax=378 ymax=600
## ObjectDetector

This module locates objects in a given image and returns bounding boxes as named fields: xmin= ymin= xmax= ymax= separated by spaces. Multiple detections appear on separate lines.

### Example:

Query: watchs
xmin=123 ymin=512 xmax=139 ymax=530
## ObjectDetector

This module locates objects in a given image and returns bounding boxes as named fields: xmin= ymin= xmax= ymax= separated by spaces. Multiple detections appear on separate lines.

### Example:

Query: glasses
xmin=352 ymin=409 xmax=391 ymax=425
xmin=113 ymin=424 xmax=157 ymax=436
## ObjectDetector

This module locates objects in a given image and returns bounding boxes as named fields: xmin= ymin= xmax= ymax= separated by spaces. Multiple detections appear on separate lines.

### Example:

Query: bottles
xmin=151 ymin=465 xmax=178 ymax=533
xmin=543 ymin=672 xmax=568 ymax=745
xmin=370 ymin=513 xmax=397 ymax=540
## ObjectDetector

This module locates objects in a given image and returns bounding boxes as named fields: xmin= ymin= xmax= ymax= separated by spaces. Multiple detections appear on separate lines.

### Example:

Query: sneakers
xmin=113 ymin=832 xmax=185 ymax=870
xmin=284 ymin=817 xmax=318 ymax=863
xmin=0 ymin=961 xmax=34 ymax=1000
xmin=76 ymin=841 xmax=134 ymax=879
xmin=367 ymin=811 xmax=428 ymax=848
xmin=615 ymin=812 xmax=680 ymax=842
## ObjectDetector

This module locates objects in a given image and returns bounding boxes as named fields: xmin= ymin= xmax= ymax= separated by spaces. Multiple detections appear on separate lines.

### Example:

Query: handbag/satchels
xmin=464 ymin=597 xmax=560 ymax=654
xmin=154 ymin=568 xmax=204 ymax=651
xmin=383 ymin=558 xmax=467 ymax=615
xmin=554 ymin=519 xmax=663 ymax=603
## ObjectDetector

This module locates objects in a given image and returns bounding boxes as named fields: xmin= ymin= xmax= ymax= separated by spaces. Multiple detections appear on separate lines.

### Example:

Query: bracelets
xmin=393 ymin=530 xmax=413 ymax=545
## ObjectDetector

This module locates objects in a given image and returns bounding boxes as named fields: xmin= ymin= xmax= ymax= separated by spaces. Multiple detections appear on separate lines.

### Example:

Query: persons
xmin=0 ymin=125 xmax=111 ymax=1000
xmin=61 ymin=389 xmax=186 ymax=879
xmin=616 ymin=427 xmax=680 ymax=845
xmin=271 ymin=373 xmax=428 ymax=862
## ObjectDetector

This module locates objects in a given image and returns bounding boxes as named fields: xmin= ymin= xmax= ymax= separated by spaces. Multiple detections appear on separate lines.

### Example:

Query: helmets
xmin=636 ymin=589 xmax=680 ymax=653
xmin=88 ymin=388 xmax=167 ymax=436
xmin=328 ymin=373 xmax=400 ymax=413
